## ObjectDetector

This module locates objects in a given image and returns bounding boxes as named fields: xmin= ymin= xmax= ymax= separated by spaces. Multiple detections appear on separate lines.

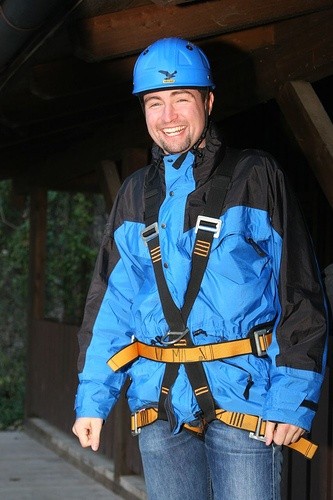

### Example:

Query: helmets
xmin=131 ymin=37 xmax=213 ymax=94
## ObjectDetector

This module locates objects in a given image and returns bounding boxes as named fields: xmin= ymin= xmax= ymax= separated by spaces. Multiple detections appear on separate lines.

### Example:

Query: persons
xmin=70 ymin=38 xmax=332 ymax=500
